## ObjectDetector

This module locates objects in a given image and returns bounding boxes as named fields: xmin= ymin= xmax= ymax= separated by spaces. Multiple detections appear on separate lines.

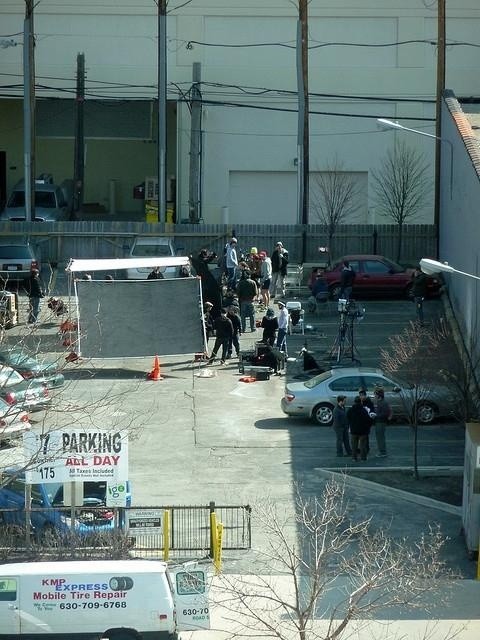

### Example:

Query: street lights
xmin=0 ymin=19 xmax=32 ymax=222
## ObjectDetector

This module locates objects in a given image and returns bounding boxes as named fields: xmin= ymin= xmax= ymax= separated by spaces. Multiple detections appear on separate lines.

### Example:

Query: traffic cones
xmin=145 ymin=353 xmax=165 ymax=381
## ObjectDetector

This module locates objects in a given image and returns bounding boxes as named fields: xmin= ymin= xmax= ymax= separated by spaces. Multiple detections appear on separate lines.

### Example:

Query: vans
xmin=0 ymin=560 xmax=213 ymax=640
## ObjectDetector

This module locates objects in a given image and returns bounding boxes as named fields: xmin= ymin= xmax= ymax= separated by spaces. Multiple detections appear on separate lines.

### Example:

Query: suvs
xmin=119 ymin=232 xmax=187 ymax=280
xmin=2 ymin=182 xmax=70 ymax=223
xmin=0 ymin=235 xmax=42 ymax=285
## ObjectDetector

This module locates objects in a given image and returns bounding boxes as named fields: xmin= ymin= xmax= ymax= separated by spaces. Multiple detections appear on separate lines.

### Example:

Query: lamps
xmin=375 ymin=118 xmax=453 ymax=204
xmin=418 ymin=257 xmax=480 ymax=290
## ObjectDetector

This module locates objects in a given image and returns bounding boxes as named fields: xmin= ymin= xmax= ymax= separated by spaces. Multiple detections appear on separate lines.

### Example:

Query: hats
xmin=230 ymin=238 xmax=238 ymax=244
xmin=251 ymin=247 xmax=258 ymax=254
xmin=278 ymin=301 xmax=285 ymax=307
xmin=259 ymin=251 xmax=266 ymax=257
xmin=276 ymin=242 xmax=283 ymax=246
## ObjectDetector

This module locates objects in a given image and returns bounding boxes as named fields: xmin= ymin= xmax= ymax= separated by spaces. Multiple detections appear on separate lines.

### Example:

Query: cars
xmin=305 ymin=254 xmax=444 ymax=304
xmin=0 ymin=396 xmax=31 ymax=446
xmin=0 ymin=364 xmax=52 ymax=414
xmin=0 ymin=460 xmax=133 ymax=544
xmin=278 ymin=364 xmax=453 ymax=425
xmin=0 ymin=340 xmax=65 ymax=391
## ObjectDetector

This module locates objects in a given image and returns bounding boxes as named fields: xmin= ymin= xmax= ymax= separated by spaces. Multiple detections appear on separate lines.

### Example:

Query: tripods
xmin=328 ymin=314 xmax=363 ymax=366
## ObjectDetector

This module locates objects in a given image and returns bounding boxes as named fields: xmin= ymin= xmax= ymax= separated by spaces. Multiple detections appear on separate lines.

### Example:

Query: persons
xmin=359 ymin=388 xmax=376 ymax=422
xmin=411 ymin=268 xmax=427 ymax=327
xmin=346 ymin=396 xmax=371 ymax=462
xmin=26 ymin=268 xmax=43 ymax=323
xmin=333 ymin=395 xmax=351 ymax=456
xmin=374 ymin=388 xmax=393 ymax=457
xmin=81 ymin=237 xmax=356 ymax=364
